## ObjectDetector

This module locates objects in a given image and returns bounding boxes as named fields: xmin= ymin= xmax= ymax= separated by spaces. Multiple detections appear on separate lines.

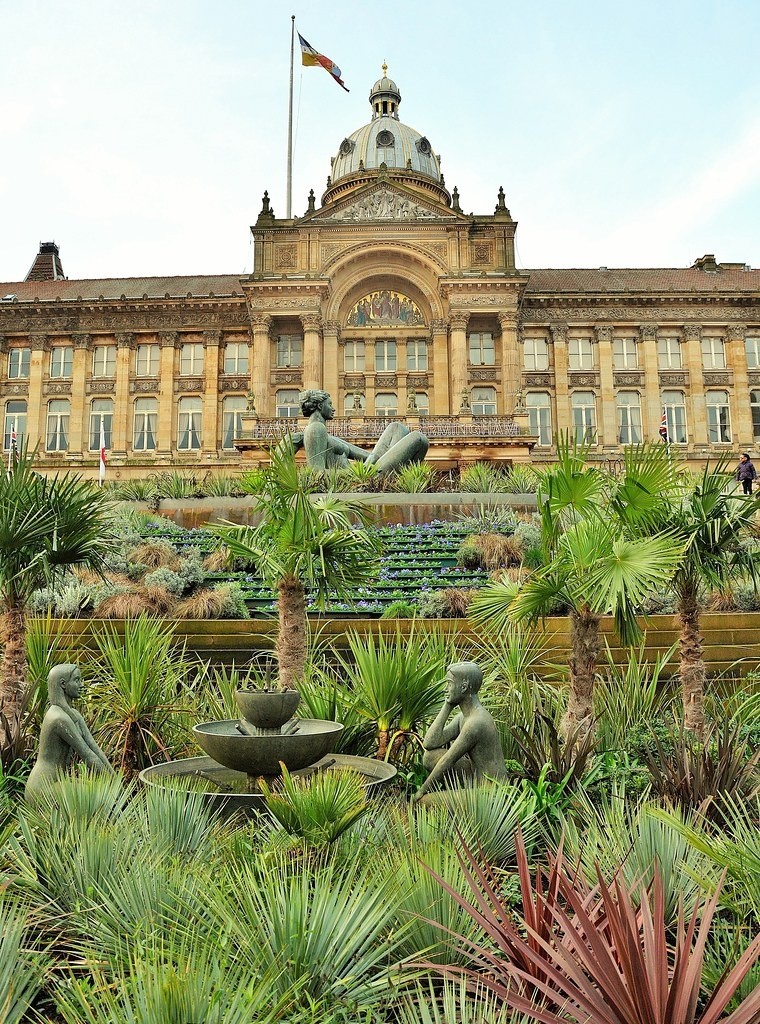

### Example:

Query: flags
xmin=659 ymin=409 xmax=666 ymax=442
xmin=99 ymin=417 xmax=106 ymax=481
xmin=12 ymin=426 xmax=19 ymax=465
xmin=297 ymin=32 xmax=349 ymax=91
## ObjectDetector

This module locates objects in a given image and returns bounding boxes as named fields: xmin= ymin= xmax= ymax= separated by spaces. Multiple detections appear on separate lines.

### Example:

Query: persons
xmin=25 ymin=664 xmax=117 ymax=807
xmin=299 ymin=389 xmax=429 ymax=482
xmin=410 ymin=662 xmax=511 ymax=813
xmin=736 ymin=453 xmax=757 ymax=495
